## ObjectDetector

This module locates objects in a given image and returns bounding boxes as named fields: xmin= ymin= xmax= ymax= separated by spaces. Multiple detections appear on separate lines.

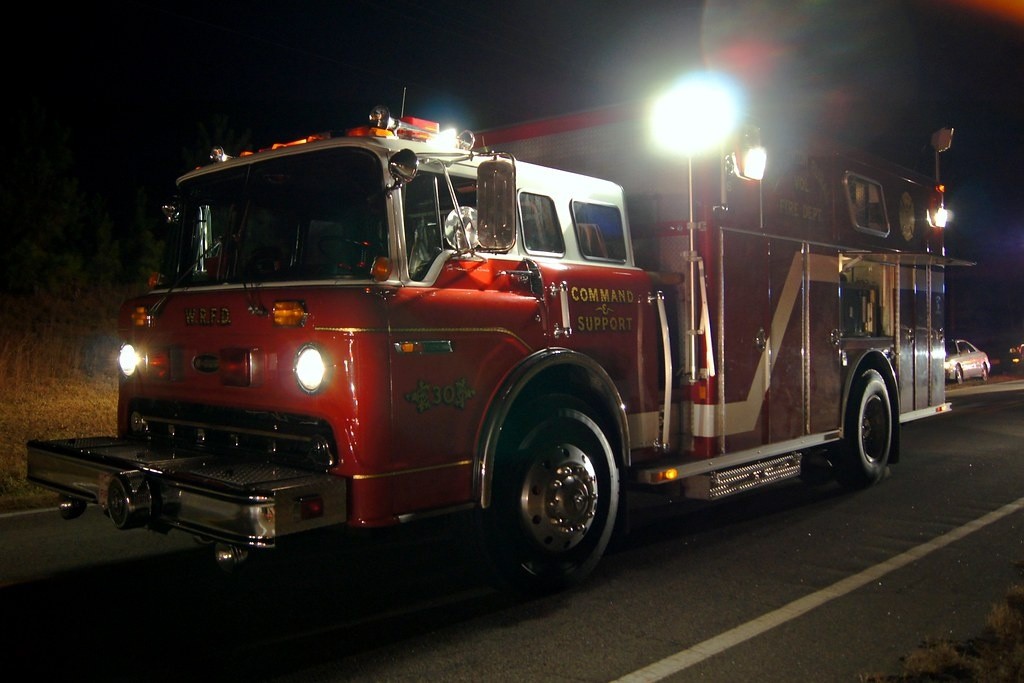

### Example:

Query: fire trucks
xmin=26 ymin=44 xmax=979 ymax=590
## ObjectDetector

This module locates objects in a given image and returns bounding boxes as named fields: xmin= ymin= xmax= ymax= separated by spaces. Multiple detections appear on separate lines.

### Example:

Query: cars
xmin=944 ymin=338 xmax=991 ymax=384
